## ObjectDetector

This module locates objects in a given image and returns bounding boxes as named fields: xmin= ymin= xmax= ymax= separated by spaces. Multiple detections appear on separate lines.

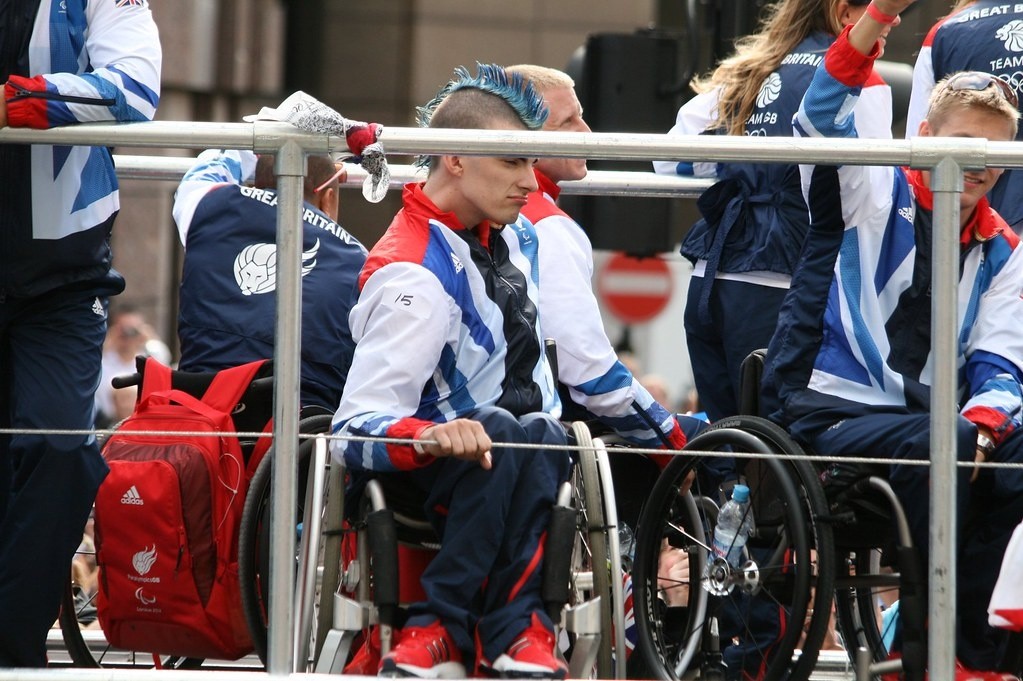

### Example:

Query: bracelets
xmin=410 ymin=420 xmax=443 ymax=455
xmin=866 ymin=0 xmax=898 ymax=26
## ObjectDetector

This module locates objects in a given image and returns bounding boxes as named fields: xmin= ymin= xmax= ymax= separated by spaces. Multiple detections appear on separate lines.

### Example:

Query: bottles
xmin=294 ymin=521 xmax=329 ymax=607
xmin=701 ymin=485 xmax=754 ymax=599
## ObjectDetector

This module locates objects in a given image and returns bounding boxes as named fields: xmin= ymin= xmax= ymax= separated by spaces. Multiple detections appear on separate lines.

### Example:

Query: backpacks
xmin=94 ymin=356 xmax=273 ymax=662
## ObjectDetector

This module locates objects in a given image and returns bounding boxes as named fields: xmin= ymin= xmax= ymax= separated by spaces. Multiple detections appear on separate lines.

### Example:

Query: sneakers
xmin=883 ymin=640 xmax=1020 ymax=681
xmin=477 ymin=609 xmax=572 ymax=681
xmin=377 ymin=609 xmax=468 ymax=679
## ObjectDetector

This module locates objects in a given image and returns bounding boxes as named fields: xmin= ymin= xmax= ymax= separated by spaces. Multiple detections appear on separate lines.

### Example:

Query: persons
xmin=82 ymin=301 xmax=177 ymax=448
xmin=329 ymin=58 xmax=582 ymax=680
xmin=172 ymin=85 xmax=379 ymax=453
xmin=484 ymin=63 xmax=787 ymax=680
xmin=0 ymin=0 xmax=166 ymax=672
xmin=760 ymin=0 xmax=1023 ymax=681
xmin=902 ymin=0 xmax=1022 ymax=258
xmin=650 ymin=0 xmax=901 ymax=649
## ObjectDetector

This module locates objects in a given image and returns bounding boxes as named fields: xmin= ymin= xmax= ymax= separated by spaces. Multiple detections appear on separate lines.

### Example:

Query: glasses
xmin=314 ymin=162 xmax=347 ymax=192
xmin=923 ymin=72 xmax=1020 ymax=121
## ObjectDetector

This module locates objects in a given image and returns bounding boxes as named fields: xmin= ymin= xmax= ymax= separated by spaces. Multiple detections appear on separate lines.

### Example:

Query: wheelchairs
xmin=632 ymin=347 xmax=1013 ymax=680
xmin=59 ymin=356 xmax=329 ymax=667
xmin=297 ymin=337 xmax=708 ymax=678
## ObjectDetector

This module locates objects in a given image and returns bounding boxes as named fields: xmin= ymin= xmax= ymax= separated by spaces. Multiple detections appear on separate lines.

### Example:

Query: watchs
xmin=972 ymin=432 xmax=994 ymax=452
